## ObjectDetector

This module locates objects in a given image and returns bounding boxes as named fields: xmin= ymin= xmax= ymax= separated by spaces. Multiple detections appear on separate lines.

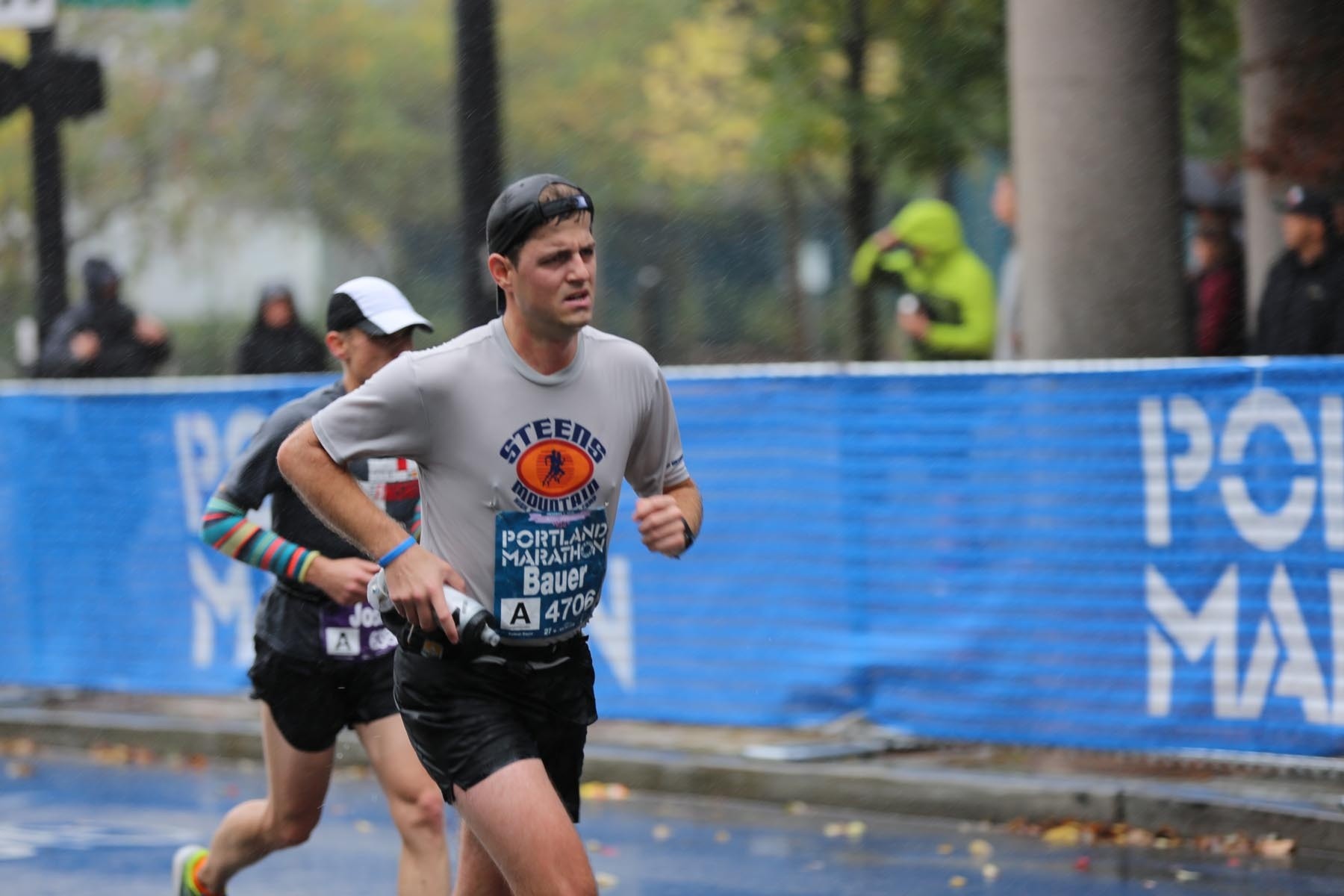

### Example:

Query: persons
xmin=848 ymin=161 xmax=1027 ymax=358
xmin=42 ymin=259 xmax=175 ymax=378
xmin=232 ymin=287 xmax=331 ymax=376
xmin=1178 ymin=179 xmax=1344 ymax=358
xmin=171 ymin=276 xmax=450 ymax=896
xmin=275 ymin=175 xmax=703 ymax=896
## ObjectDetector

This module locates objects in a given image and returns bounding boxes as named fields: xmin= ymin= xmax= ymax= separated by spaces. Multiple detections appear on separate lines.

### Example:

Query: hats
xmin=1272 ymin=183 xmax=1327 ymax=215
xmin=327 ymin=276 xmax=434 ymax=339
xmin=487 ymin=173 xmax=594 ymax=315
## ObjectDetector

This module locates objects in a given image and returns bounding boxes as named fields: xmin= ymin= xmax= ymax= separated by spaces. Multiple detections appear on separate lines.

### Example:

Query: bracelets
xmin=678 ymin=515 xmax=695 ymax=559
xmin=377 ymin=535 xmax=418 ymax=568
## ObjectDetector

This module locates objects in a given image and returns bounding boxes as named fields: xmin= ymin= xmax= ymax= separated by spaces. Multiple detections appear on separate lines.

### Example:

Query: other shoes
xmin=173 ymin=846 xmax=226 ymax=896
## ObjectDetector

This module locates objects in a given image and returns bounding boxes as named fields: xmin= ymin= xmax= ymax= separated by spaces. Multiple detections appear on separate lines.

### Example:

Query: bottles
xmin=367 ymin=572 xmax=395 ymax=612
xmin=377 ymin=566 xmax=501 ymax=647
